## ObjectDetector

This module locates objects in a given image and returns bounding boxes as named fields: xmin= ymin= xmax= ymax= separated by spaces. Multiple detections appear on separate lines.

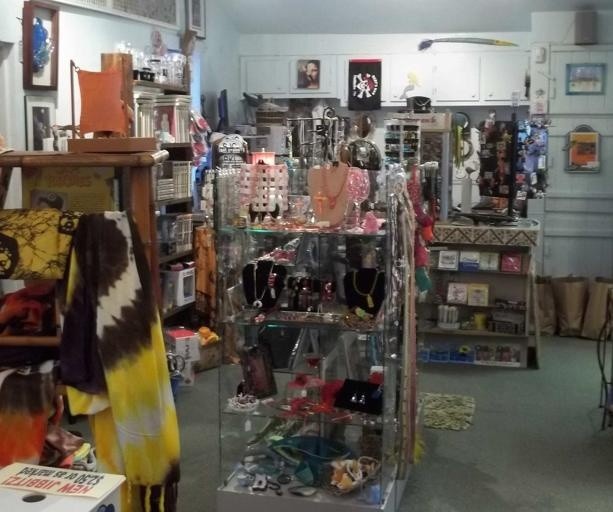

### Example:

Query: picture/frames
xmin=565 ymin=63 xmax=606 ymax=95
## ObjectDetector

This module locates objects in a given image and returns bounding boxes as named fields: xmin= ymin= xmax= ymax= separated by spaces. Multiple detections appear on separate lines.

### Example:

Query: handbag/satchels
xmin=534 ymin=273 xmax=613 ymax=341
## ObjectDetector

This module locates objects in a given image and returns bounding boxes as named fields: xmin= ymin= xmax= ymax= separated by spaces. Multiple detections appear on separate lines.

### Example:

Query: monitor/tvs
xmin=215 ymin=89 xmax=228 ymax=132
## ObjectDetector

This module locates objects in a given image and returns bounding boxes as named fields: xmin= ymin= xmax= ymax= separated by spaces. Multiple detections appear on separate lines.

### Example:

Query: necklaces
xmin=250 ymin=260 xmax=273 ymax=314
xmin=316 ymin=166 xmax=348 ymax=211
xmin=352 ymin=271 xmax=378 ymax=310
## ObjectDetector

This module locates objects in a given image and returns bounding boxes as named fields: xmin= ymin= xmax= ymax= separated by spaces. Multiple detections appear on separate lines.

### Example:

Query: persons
xmin=337 ymin=266 xmax=386 ymax=320
xmin=298 ymin=60 xmax=319 ymax=89
xmin=237 ymin=259 xmax=288 ymax=314
xmin=307 ymin=159 xmax=355 ymax=226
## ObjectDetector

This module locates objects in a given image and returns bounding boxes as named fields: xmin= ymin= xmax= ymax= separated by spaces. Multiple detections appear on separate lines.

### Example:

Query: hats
xmin=258 ymin=103 xmax=288 ymax=112
xmin=453 ymin=112 xmax=470 ymax=133
xmin=399 ymin=97 xmax=430 ymax=112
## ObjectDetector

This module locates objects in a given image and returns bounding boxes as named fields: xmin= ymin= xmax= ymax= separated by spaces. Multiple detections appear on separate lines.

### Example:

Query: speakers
xmin=575 ymin=11 xmax=597 ymax=44
